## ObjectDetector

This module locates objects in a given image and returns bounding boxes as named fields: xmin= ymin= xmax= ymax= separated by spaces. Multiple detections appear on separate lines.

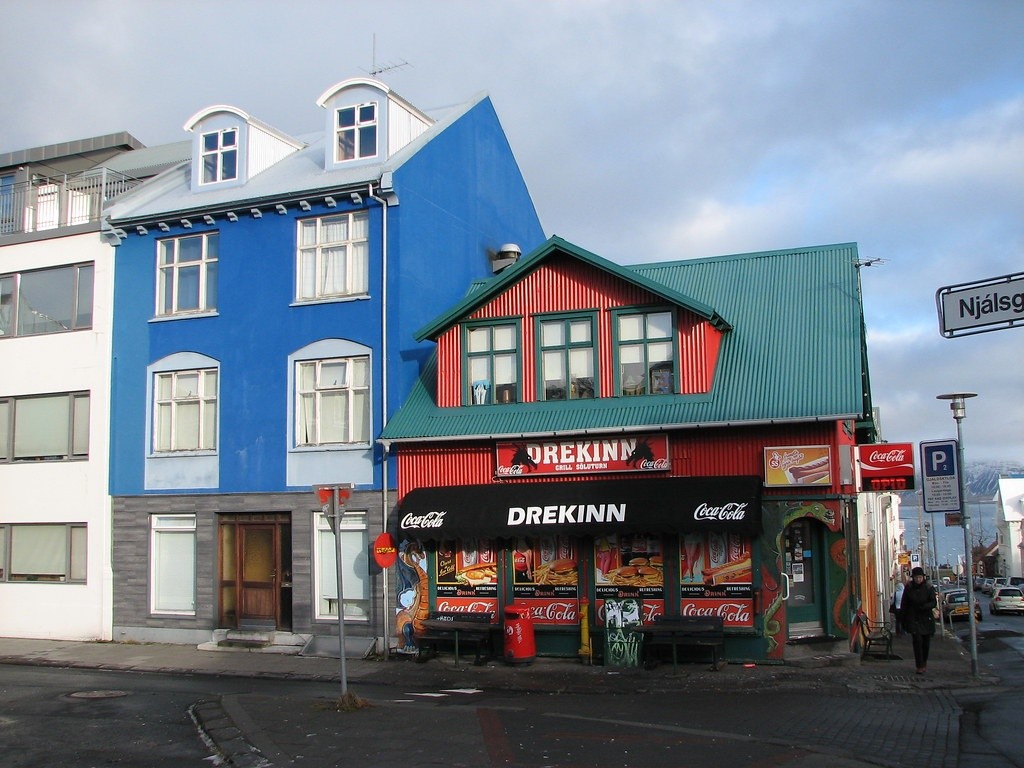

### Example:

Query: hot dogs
xmin=789 ymin=456 xmax=829 ymax=478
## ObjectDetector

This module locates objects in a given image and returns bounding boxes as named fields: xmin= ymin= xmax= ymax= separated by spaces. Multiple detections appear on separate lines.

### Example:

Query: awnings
xmin=396 ymin=475 xmax=764 ymax=544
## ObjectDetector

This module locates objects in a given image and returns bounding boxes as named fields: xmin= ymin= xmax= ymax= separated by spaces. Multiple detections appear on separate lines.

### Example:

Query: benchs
xmin=857 ymin=611 xmax=895 ymax=661
xmin=648 ymin=613 xmax=726 ymax=671
xmin=416 ymin=610 xmax=495 ymax=667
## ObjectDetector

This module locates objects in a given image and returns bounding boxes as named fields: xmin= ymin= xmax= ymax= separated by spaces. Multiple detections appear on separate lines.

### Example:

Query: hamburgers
xmin=455 ymin=563 xmax=497 ymax=584
xmin=549 ymin=558 xmax=576 ymax=576
xmin=616 ymin=556 xmax=663 ymax=585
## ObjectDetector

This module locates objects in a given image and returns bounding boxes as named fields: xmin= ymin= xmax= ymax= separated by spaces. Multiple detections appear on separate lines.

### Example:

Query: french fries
xmin=533 ymin=564 xmax=578 ymax=585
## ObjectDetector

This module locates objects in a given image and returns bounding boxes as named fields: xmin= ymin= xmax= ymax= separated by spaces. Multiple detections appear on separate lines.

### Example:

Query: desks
xmin=419 ymin=618 xmax=493 ymax=674
xmin=632 ymin=624 xmax=715 ymax=679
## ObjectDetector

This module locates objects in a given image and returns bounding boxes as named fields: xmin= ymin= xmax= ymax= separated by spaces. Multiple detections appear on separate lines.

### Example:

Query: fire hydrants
xmin=577 ymin=597 xmax=590 ymax=655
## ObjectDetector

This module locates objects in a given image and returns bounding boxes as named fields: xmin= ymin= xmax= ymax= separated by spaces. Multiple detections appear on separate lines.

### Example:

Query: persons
xmin=901 ymin=567 xmax=936 ymax=673
xmin=892 ymin=582 xmax=905 ymax=636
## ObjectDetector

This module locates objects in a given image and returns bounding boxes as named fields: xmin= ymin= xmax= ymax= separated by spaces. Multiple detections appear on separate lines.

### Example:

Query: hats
xmin=910 ymin=567 xmax=926 ymax=578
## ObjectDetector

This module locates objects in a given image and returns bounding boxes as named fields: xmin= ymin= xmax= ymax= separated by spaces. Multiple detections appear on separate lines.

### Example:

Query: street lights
xmin=936 ymin=392 xmax=979 ymax=681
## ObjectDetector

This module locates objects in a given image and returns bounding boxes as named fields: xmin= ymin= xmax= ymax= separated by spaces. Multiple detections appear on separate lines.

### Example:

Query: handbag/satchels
xmin=889 ymin=602 xmax=896 ymax=613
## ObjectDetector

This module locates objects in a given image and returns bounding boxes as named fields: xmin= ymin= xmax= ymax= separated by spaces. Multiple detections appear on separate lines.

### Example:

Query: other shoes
xmin=917 ymin=660 xmax=927 ymax=674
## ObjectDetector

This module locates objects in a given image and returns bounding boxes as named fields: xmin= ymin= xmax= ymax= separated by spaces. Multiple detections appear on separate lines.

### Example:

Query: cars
xmin=1002 ymin=576 xmax=1024 ymax=594
xmin=989 ymin=587 xmax=1024 ymax=616
xmin=942 ymin=592 xmax=983 ymax=624
xmin=941 ymin=589 xmax=968 ymax=600
xmin=929 ymin=577 xmax=1006 ymax=596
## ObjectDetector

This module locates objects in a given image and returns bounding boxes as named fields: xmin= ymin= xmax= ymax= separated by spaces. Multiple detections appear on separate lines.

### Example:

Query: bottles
xmin=742 ymin=664 xmax=755 ymax=668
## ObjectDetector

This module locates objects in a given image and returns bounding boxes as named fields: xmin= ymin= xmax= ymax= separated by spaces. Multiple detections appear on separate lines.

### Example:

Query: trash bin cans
xmin=503 ymin=603 xmax=537 ymax=663
